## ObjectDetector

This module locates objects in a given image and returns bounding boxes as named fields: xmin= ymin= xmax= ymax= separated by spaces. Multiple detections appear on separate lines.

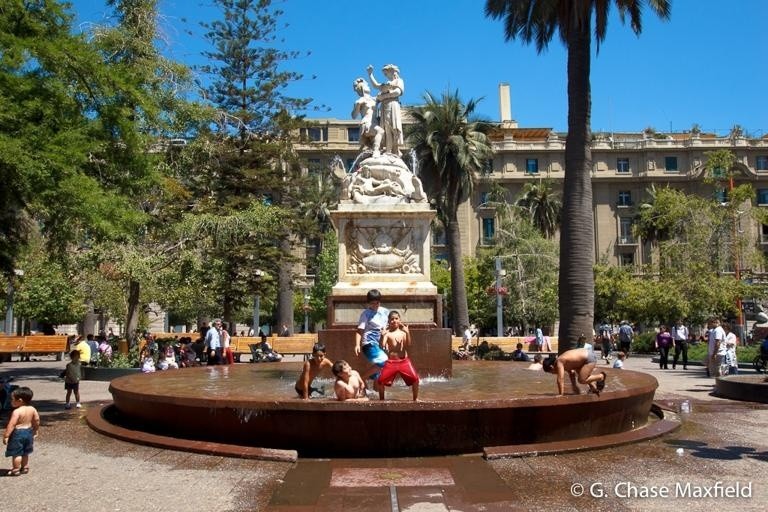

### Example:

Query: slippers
xmin=596 ymin=372 xmax=606 ymax=391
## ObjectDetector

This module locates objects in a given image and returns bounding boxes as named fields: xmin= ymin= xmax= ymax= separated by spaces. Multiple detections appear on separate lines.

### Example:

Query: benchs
xmin=451 ymin=335 xmax=559 ymax=357
xmin=148 ymin=332 xmax=318 ymax=362
xmin=1 ymin=334 xmax=68 ymax=361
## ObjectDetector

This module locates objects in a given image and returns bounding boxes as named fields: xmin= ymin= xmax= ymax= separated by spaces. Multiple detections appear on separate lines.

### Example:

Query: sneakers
xmin=64 ymin=402 xmax=81 ymax=410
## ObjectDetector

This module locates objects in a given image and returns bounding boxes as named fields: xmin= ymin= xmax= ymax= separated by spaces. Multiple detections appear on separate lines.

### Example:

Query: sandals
xmin=20 ymin=467 xmax=29 ymax=475
xmin=4 ymin=469 xmax=21 ymax=477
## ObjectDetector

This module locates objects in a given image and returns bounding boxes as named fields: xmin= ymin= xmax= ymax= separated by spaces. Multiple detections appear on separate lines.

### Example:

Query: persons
xmin=355 ymin=165 xmax=411 ymax=200
xmin=528 ymin=353 xmax=543 ymax=371
xmin=458 ymin=324 xmax=472 ymax=350
xmin=535 ymin=323 xmax=544 ymax=352
xmin=294 ymin=344 xmax=334 ymax=400
xmin=353 ymin=78 xmax=386 ymax=156
xmin=58 ymin=320 xmax=290 ymax=381
xmin=613 ymin=352 xmax=625 ymax=369
xmin=576 ymin=334 xmax=592 ymax=351
xmin=59 ymin=349 xmax=81 ymax=410
xmin=760 ymin=335 xmax=768 ymax=364
xmin=543 ymin=348 xmax=606 ymax=397
xmin=702 ymin=316 xmax=738 ymax=397
xmin=366 ymin=63 xmax=405 ymax=159
xmin=594 ymin=318 xmax=634 ymax=364
xmin=654 ymin=324 xmax=672 ymax=369
xmin=332 ymin=359 xmax=366 ymax=400
xmin=512 ymin=343 xmax=531 ymax=361
xmin=378 ymin=311 xmax=419 ymax=401
xmin=671 ymin=318 xmax=689 ymax=370
xmin=354 ymin=289 xmax=389 ymax=391
xmin=3 ymin=386 xmax=41 ymax=476
